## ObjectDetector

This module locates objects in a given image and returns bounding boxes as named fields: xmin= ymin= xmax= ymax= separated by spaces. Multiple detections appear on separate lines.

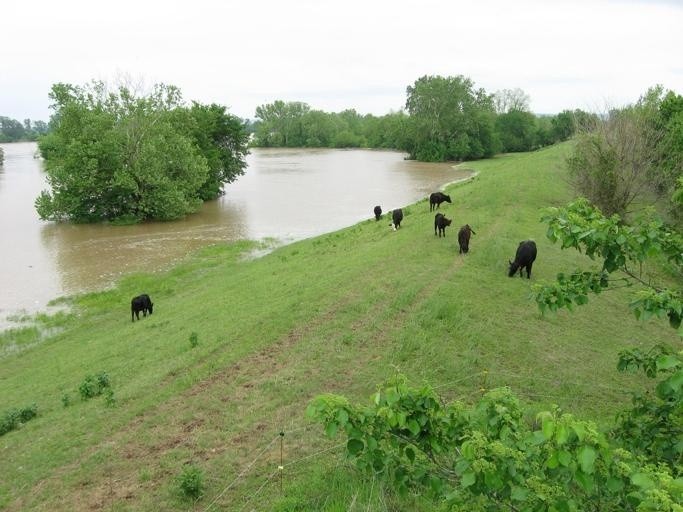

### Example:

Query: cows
xmin=392 ymin=208 xmax=403 ymax=231
xmin=374 ymin=206 xmax=382 ymax=221
xmin=435 ymin=212 xmax=451 ymax=237
xmin=458 ymin=224 xmax=476 ymax=254
xmin=508 ymin=240 xmax=537 ymax=279
xmin=131 ymin=294 xmax=153 ymax=322
xmin=430 ymin=192 xmax=452 ymax=211
